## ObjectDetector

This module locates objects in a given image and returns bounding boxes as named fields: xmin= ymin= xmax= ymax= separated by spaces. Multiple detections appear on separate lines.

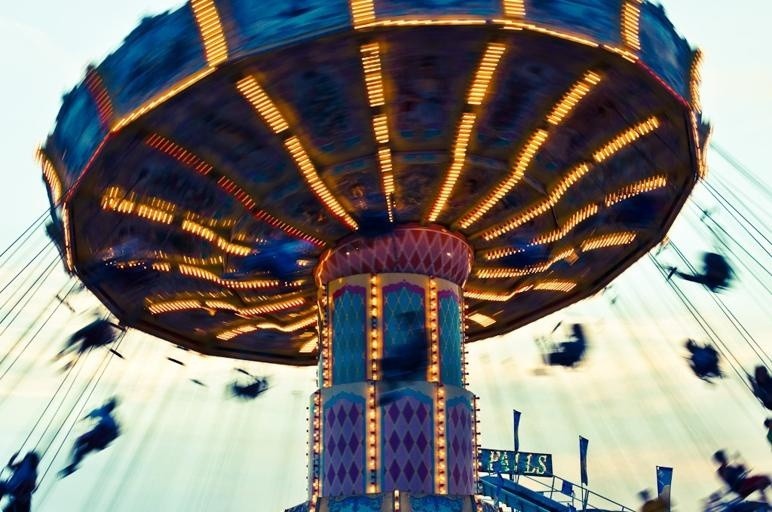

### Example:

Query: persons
xmin=0 ymin=227 xmax=772 ymax=511
